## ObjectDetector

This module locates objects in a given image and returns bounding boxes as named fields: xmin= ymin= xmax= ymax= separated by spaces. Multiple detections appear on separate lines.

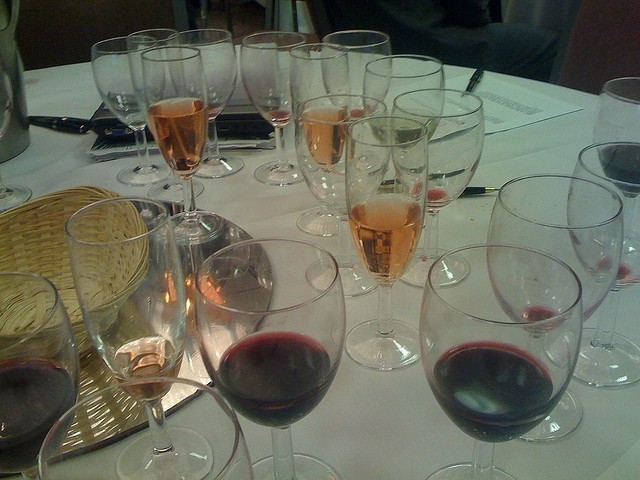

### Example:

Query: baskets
xmin=0 ymin=185 xmax=151 ymax=364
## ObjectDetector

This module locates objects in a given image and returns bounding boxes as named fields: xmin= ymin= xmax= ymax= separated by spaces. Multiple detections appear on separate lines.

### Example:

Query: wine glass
xmin=294 ymin=94 xmax=390 ymax=299
xmin=166 ymin=29 xmax=244 ymax=180
xmin=417 ymin=242 xmax=584 ymax=480
xmin=36 ymin=378 xmax=254 ymax=480
xmin=66 ymin=195 xmax=215 ymax=479
xmin=238 ymin=31 xmax=306 ymax=187
xmin=141 ymin=45 xmax=224 ymax=247
xmin=126 ymin=28 xmax=205 ymax=202
xmin=389 ymin=89 xmax=485 ymax=288
xmin=595 ymin=77 xmax=640 ymax=287
xmin=90 ymin=35 xmax=171 ymax=187
xmin=321 ymin=29 xmax=392 ymax=172
xmin=289 ymin=43 xmax=353 ymax=236
xmin=0 ymin=69 xmax=33 ymax=213
xmin=485 ymin=172 xmax=623 ymax=444
xmin=361 ymin=54 xmax=445 ymax=151
xmin=566 ymin=141 xmax=640 ymax=388
xmin=0 ymin=272 xmax=80 ymax=479
xmin=344 ymin=115 xmax=439 ymax=371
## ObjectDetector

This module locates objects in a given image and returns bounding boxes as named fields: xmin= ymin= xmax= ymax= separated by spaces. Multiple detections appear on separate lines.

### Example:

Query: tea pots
xmin=194 ymin=237 xmax=345 ymax=480
xmin=0 ymin=0 xmax=31 ymax=164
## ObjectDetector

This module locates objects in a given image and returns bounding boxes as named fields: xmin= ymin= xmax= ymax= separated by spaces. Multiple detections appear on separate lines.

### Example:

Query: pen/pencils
xmin=462 ymin=186 xmax=500 ymax=195
xmin=461 ymin=68 xmax=484 ymax=98
xmin=29 ymin=116 xmax=90 ymax=134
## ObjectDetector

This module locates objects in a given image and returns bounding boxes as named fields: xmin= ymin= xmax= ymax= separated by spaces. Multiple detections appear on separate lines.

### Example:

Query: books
xmin=85 ymin=127 xmax=286 ymax=163
